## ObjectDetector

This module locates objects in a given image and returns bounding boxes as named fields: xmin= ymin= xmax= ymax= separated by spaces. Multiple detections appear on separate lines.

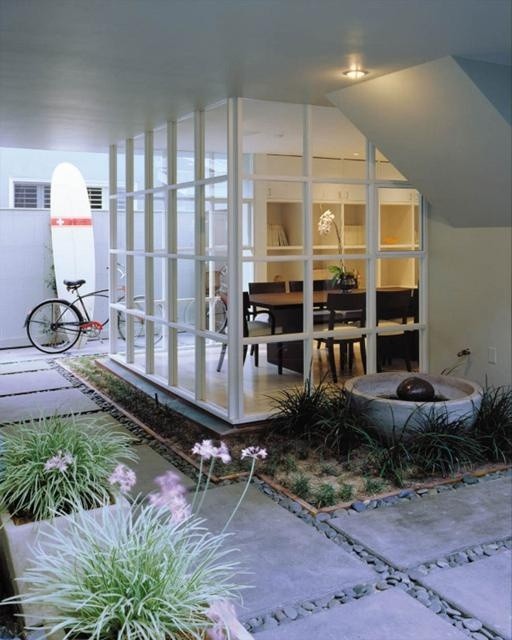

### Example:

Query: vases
xmin=337 ymin=271 xmax=356 ymax=293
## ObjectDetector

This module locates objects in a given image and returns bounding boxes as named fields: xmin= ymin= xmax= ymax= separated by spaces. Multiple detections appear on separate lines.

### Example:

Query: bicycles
xmin=184 ymin=264 xmax=227 ymax=332
xmin=23 ymin=261 xmax=164 ymax=353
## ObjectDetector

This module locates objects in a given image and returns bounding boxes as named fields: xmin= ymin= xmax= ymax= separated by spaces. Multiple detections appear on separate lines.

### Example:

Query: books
xmin=266 ymin=223 xmax=288 ymax=247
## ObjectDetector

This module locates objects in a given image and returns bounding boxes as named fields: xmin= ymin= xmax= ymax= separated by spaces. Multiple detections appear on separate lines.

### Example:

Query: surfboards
xmin=48 ymin=161 xmax=96 ymax=349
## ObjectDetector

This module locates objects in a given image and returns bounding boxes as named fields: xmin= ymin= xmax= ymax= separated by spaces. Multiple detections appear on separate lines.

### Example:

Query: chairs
xmin=216 ymin=279 xmax=419 ymax=383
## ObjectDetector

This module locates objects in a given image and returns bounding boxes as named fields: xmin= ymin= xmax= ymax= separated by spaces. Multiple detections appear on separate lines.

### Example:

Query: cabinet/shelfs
xmin=265 ymin=198 xmax=420 ymax=326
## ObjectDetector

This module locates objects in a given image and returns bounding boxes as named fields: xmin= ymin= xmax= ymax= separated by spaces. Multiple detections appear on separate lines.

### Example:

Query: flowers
xmin=317 ymin=209 xmax=345 ymax=287
